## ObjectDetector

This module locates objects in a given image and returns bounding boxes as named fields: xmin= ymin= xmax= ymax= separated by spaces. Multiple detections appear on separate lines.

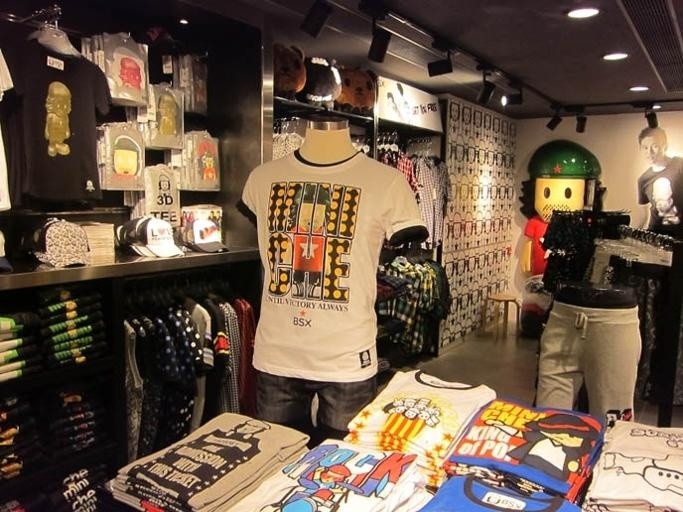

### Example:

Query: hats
xmin=0 ymin=230 xmax=13 ymax=270
xmin=173 ymin=218 xmax=230 ymax=253
xmin=113 ymin=216 xmax=187 ymax=258
xmin=21 ymin=217 xmax=90 ymax=268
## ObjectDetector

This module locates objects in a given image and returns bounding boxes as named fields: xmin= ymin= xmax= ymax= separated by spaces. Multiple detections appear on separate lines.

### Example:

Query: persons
xmin=520 ymin=138 xmax=605 ymax=337
xmin=637 ymin=126 xmax=683 ymax=243
xmin=535 ymin=287 xmax=641 ymax=425
xmin=238 ymin=120 xmax=430 ymax=433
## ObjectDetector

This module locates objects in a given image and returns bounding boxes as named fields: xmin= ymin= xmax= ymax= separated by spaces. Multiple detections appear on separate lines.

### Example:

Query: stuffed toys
xmin=271 ymin=40 xmax=376 ymax=117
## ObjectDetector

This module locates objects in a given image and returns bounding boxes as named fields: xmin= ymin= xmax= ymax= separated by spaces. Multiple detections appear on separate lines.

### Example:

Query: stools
xmin=480 ymin=292 xmax=521 ymax=345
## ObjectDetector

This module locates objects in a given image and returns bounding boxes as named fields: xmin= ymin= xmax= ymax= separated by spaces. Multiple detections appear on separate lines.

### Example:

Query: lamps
xmin=576 ymin=107 xmax=589 ymax=133
xmin=307 ymin=2 xmax=335 ymax=38
xmin=644 ymin=103 xmax=658 ymax=130
xmin=546 ymin=103 xmax=563 ymax=131
xmin=366 ymin=28 xmax=391 ymax=63
xmin=475 ymin=72 xmax=496 ymax=107
xmin=425 ymin=51 xmax=453 ymax=77
xmin=499 ymin=84 xmax=524 ymax=108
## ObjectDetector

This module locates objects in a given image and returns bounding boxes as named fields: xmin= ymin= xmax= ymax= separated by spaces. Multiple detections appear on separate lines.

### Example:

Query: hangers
xmin=276 ymin=117 xmax=436 ymax=160
xmin=26 ymin=7 xmax=81 ymax=59
xmin=600 ymin=227 xmax=675 ymax=273
xmin=121 ymin=266 xmax=234 ymax=319
